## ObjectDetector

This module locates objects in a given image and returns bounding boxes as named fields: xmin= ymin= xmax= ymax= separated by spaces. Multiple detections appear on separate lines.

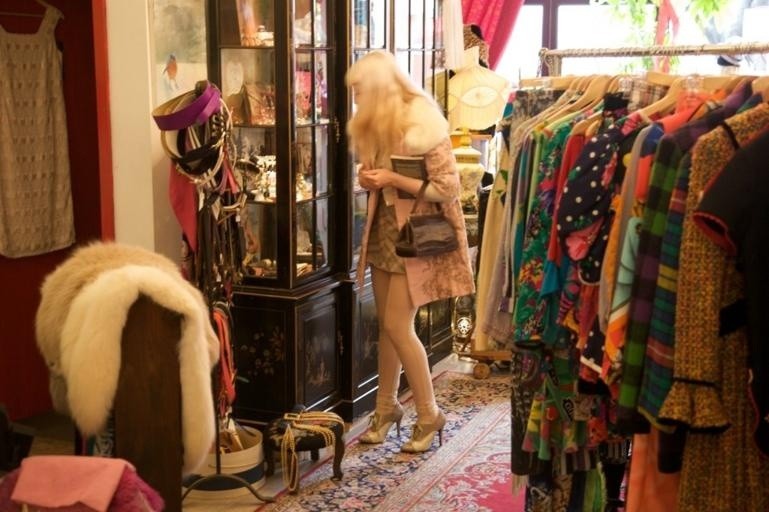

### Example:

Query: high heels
xmin=402 ymin=409 xmax=446 ymax=452
xmin=357 ymin=402 xmax=403 ymax=443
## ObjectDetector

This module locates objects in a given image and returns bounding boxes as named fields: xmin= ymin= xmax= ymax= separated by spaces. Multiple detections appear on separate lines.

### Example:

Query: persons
xmin=344 ymin=49 xmax=477 ymax=452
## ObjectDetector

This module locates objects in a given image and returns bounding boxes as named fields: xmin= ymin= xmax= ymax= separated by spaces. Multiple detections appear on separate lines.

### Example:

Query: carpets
xmin=252 ymin=370 xmax=526 ymax=511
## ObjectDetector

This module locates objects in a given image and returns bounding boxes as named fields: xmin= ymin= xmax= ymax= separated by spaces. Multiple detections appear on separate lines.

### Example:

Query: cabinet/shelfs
xmin=203 ymin=0 xmax=455 ymax=433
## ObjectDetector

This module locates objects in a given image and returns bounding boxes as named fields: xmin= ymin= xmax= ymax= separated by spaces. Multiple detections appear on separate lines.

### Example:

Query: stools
xmin=265 ymin=411 xmax=345 ymax=485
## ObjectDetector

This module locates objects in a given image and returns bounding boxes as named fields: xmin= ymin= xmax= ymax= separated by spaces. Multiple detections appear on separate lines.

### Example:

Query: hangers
xmin=1 ymin=0 xmax=68 ymax=20
xmin=534 ymin=45 xmax=689 ymax=139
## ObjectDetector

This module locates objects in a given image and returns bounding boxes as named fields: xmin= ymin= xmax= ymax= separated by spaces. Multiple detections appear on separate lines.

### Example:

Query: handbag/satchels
xmin=395 ymin=214 xmax=458 ymax=257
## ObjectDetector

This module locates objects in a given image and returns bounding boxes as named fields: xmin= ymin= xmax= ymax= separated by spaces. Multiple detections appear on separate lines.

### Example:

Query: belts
xmin=277 ymin=404 xmax=306 ymax=434
xmin=153 ymin=83 xmax=246 ymax=418
